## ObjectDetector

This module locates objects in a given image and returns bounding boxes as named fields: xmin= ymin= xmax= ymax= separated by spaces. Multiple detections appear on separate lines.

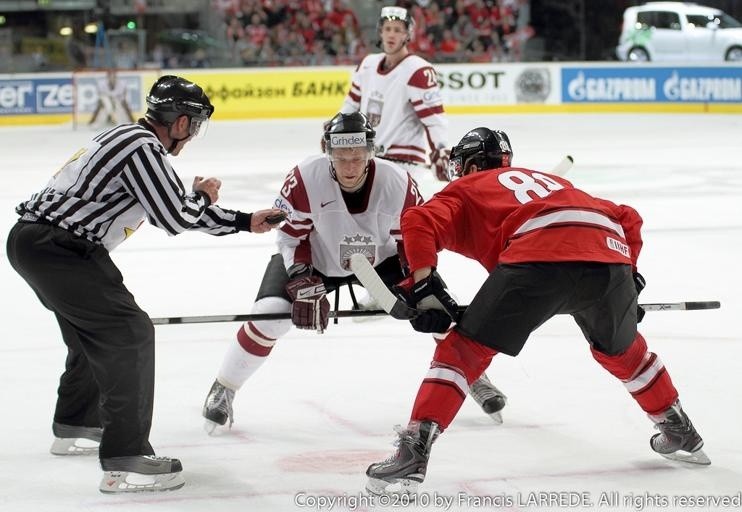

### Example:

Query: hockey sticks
xmin=348 ymin=253 xmax=720 ymax=319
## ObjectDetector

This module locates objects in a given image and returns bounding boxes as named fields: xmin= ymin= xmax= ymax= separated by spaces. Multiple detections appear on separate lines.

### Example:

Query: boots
xmin=644 ymin=396 xmax=715 ymax=468
xmin=365 ymin=417 xmax=442 ymax=502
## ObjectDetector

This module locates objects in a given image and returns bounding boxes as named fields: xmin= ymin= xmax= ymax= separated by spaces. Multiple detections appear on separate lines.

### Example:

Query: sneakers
xmin=466 ymin=369 xmax=504 ymax=422
xmin=93 ymin=440 xmax=185 ymax=494
xmin=50 ymin=411 xmax=123 ymax=457
xmin=200 ymin=372 xmax=237 ymax=433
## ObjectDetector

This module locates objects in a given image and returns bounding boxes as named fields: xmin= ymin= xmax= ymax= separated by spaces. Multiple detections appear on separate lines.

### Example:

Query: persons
xmin=71 ymin=1 xmax=532 ymax=72
xmin=4 ymin=73 xmax=289 ymax=476
xmin=339 ymin=3 xmax=454 ymax=183
xmin=202 ymin=111 xmax=509 ymax=427
xmin=366 ymin=126 xmax=705 ymax=484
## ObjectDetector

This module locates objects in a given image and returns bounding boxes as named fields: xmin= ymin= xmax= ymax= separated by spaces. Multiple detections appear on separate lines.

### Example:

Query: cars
xmin=612 ymin=1 xmax=742 ymax=66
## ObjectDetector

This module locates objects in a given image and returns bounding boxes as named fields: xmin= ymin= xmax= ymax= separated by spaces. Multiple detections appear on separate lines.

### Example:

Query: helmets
xmin=447 ymin=126 xmax=513 ymax=182
xmin=320 ymin=111 xmax=378 ymax=163
xmin=144 ymin=73 xmax=213 ymax=151
xmin=373 ymin=7 xmax=415 ymax=53
